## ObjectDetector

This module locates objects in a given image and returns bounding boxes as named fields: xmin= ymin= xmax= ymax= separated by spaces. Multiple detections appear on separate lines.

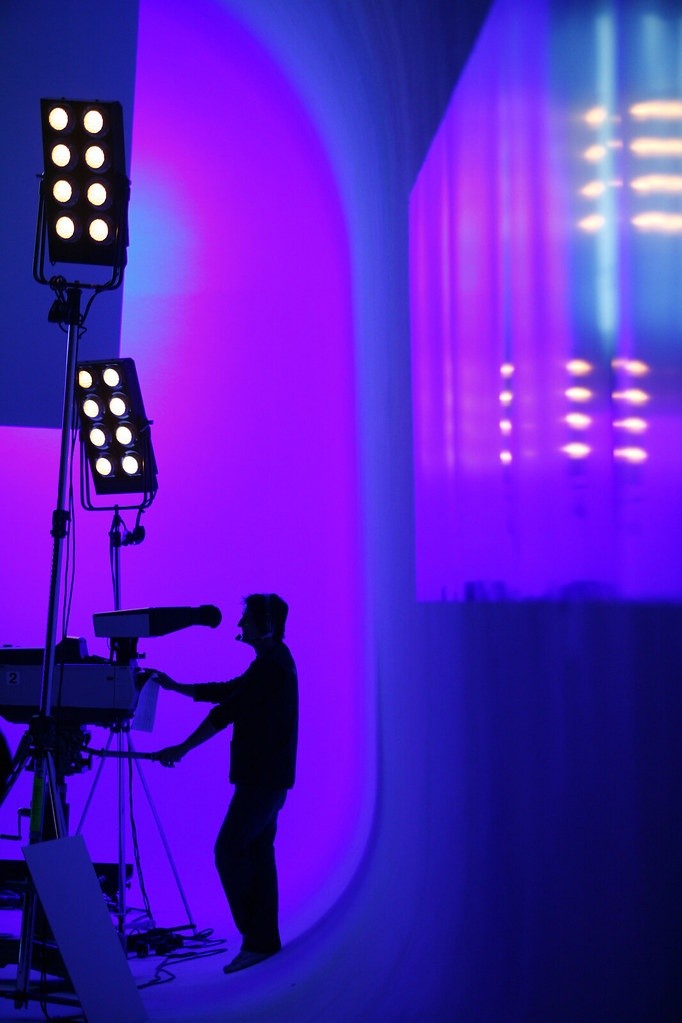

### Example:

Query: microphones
xmin=234 ymin=634 xmax=242 ymax=641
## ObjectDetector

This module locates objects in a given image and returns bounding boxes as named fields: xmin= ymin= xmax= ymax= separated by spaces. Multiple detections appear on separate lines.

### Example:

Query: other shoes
xmin=223 ymin=949 xmax=263 ymax=974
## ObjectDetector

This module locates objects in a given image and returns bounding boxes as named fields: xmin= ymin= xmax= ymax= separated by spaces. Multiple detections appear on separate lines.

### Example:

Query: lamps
xmin=32 ymin=97 xmax=132 ymax=329
xmin=75 ymin=358 xmax=159 ymax=546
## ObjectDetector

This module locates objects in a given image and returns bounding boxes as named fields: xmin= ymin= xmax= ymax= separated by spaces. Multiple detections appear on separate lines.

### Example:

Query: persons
xmin=154 ymin=593 xmax=301 ymax=971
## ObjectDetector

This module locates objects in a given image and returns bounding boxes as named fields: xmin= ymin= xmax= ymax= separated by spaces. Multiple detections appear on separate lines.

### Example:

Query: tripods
xmin=77 ymin=727 xmax=197 ymax=937
